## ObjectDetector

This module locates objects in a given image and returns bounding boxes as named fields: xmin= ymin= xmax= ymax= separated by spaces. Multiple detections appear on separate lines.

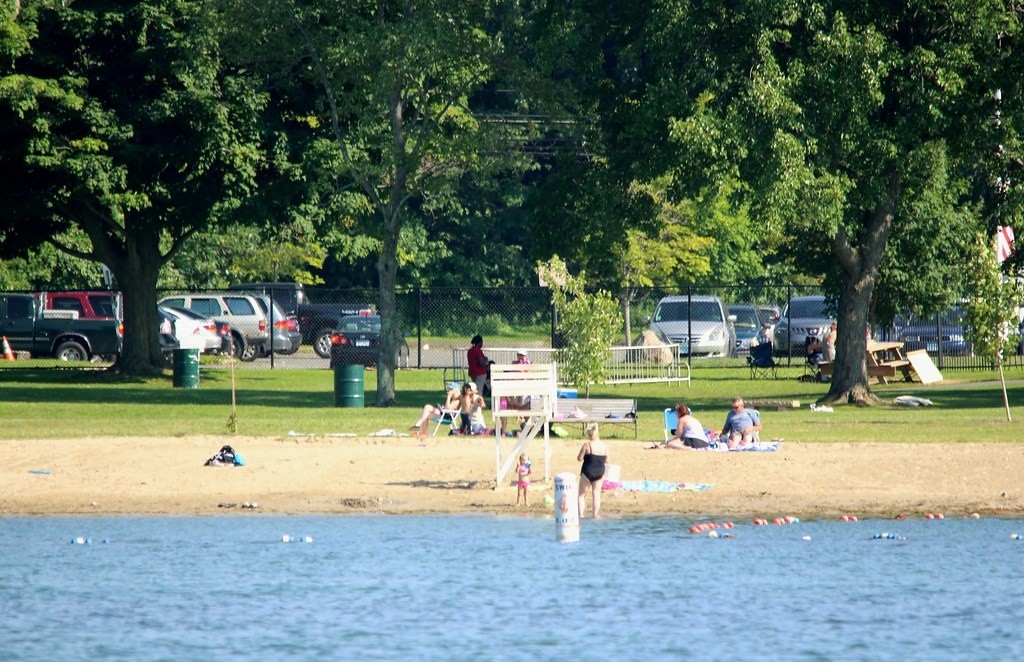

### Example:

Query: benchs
xmin=879 ymin=359 xmax=909 ymax=368
xmin=530 ymin=397 xmax=638 ymax=439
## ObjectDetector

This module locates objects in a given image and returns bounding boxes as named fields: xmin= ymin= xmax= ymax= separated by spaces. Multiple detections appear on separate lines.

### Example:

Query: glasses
xmin=732 ymin=405 xmax=741 ymax=409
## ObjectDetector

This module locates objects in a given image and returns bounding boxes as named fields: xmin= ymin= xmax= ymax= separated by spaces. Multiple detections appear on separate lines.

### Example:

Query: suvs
xmin=727 ymin=305 xmax=780 ymax=352
xmin=251 ymin=293 xmax=303 ymax=359
xmin=772 ymin=295 xmax=835 ymax=351
xmin=646 ymin=293 xmax=738 ymax=361
xmin=157 ymin=292 xmax=269 ymax=362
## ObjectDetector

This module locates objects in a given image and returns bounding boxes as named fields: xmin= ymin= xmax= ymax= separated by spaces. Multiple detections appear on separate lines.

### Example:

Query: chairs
xmin=663 ymin=408 xmax=692 ymax=442
xmin=373 ymin=324 xmax=381 ymax=332
xmin=805 ymin=336 xmax=820 ymax=376
xmin=429 ymin=406 xmax=462 ymax=436
xmin=483 ymin=381 xmax=491 ymax=398
xmin=746 ymin=342 xmax=783 ymax=380
xmin=346 ymin=323 xmax=358 ymax=332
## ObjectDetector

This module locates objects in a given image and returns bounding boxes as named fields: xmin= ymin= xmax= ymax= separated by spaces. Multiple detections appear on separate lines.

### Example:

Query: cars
xmin=899 ymin=296 xmax=970 ymax=358
xmin=31 ymin=291 xmax=235 ymax=370
xmin=328 ymin=313 xmax=410 ymax=369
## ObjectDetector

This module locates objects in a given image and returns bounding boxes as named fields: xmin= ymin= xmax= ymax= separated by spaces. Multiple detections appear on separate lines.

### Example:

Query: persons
xmin=576 ymin=423 xmax=609 ymax=518
xmin=515 ymin=454 xmax=531 ymax=507
xmin=665 ymin=403 xmax=709 ymax=451
xmin=804 ymin=320 xmax=836 ymax=368
xmin=717 ymin=397 xmax=762 ymax=449
xmin=483 ymin=360 xmax=496 ymax=397
xmin=409 ymin=380 xmax=487 ymax=437
xmin=499 ymin=394 xmax=554 ymax=437
xmin=467 ymin=334 xmax=489 ymax=396
xmin=512 ymin=349 xmax=532 ymax=373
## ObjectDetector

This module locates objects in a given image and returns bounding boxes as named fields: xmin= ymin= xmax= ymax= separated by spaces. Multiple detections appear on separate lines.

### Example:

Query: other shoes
xmin=409 ymin=426 xmax=419 ymax=431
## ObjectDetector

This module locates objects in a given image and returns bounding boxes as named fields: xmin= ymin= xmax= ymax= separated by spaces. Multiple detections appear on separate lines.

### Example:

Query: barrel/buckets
xmin=335 ymin=365 xmax=363 ymax=407
xmin=172 ymin=349 xmax=199 ymax=388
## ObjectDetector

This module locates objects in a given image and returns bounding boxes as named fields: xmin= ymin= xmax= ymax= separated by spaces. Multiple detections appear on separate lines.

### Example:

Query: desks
xmin=818 ymin=342 xmax=913 ymax=385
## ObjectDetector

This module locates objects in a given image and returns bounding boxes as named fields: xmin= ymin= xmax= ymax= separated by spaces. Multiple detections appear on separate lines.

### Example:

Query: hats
xmin=517 ymin=348 xmax=526 ymax=356
xmin=447 ymin=383 xmax=459 ymax=389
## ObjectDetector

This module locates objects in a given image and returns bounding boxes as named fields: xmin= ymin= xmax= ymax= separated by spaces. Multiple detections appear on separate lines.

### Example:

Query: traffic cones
xmin=1 ymin=335 xmax=16 ymax=362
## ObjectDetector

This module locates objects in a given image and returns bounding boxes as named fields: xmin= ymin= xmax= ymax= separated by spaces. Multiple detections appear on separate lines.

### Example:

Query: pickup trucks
xmin=228 ymin=281 xmax=372 ymax=359
xmin=0 ymin=291 xmax=124 ymax=364
